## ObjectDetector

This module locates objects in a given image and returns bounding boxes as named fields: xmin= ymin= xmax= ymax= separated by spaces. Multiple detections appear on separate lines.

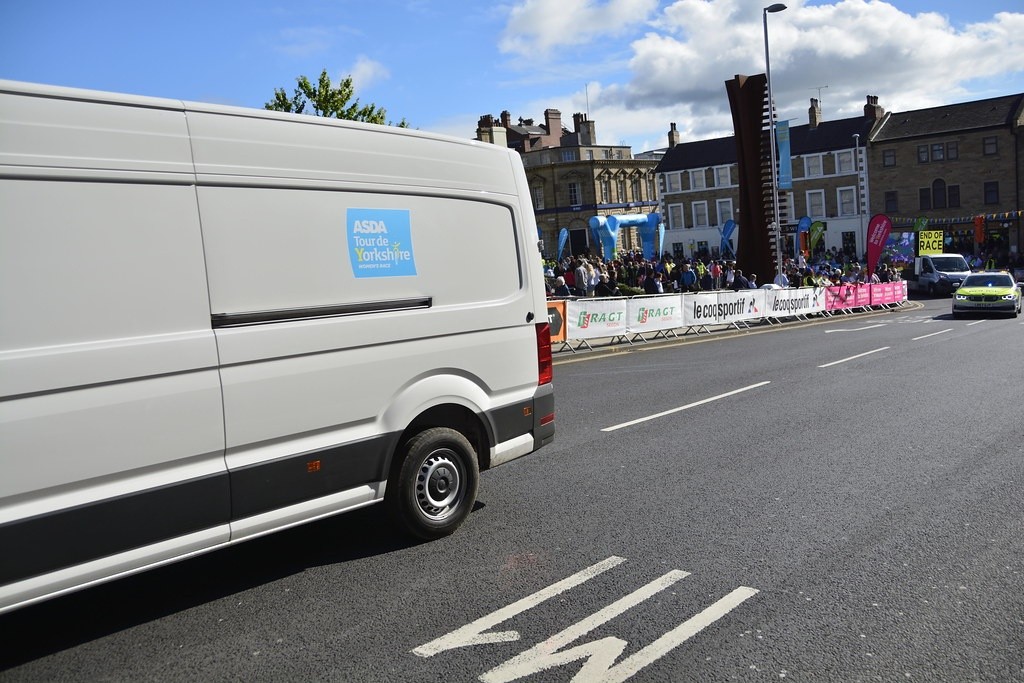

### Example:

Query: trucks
xmin=902 ymin=254 xmax=972 ymax=296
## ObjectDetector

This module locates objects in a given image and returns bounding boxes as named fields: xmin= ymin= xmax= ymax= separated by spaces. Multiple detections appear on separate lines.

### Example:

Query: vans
xmin=0 ymin=81 xmax=554 ymax=614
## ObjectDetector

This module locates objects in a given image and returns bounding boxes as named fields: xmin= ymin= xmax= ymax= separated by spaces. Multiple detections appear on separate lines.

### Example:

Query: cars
xmin=950 ymin=272 xmax=1024 ymax=316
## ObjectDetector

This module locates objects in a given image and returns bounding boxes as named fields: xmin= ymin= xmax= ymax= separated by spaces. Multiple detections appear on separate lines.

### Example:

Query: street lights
xmin=853 ymin=134 xmax=864 ymax=259
xmin=763 ymin=3 xmax=789 ymax=286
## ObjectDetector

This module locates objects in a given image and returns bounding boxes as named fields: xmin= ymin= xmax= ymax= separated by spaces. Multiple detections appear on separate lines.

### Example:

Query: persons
xmin=774 ymin=244 xmax=903 ymax=322
xmin=726 ymin=265 xmax=735 ymax=290
xmin=748 ymin=274 xmax=757 ymax=289
xmin=732 ymin=269 xmax=750 ymax=292
xmin=961 ymin=246 xmax=1024 ymax=270
xmin=542 ymin=247 xmax=735 ymax=301
